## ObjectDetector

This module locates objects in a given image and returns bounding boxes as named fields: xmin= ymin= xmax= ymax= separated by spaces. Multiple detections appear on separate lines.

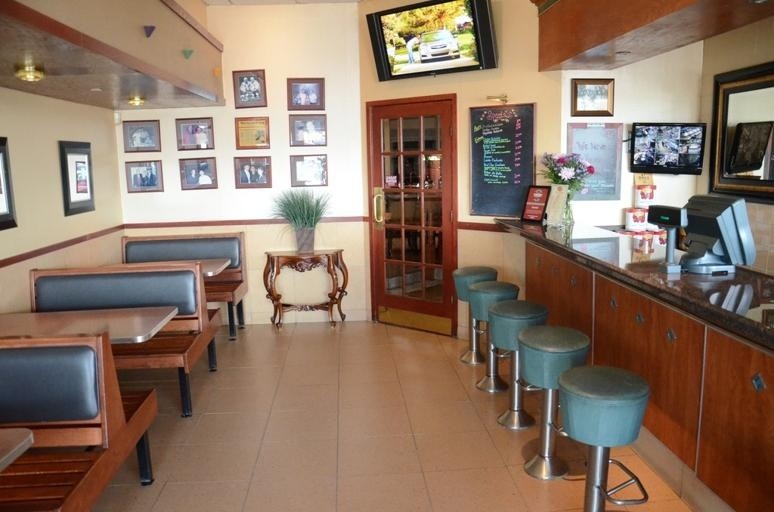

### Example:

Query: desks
xmin=494 ymin=219 xmax=773 ymax=511
xmin=264 ymin=250 xmax=347 ymax=329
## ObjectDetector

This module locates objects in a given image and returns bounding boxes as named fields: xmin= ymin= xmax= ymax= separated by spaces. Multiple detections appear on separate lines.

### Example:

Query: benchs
xmin=33 ymin=262 xmax=223 ymax=371
xmin=121 ymin=232 xmax=247 ymax=341
xmin=0 ymin=339 xmax=111 ymax=512
xmin=0 ymin=334 xmax=159 ymax=485
xmin=30 ymin=263 xmax=207 ymax=417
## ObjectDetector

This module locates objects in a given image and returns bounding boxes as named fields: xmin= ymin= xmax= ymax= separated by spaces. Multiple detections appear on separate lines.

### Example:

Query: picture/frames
xmin=707 ymin=60 xmax=774 ymax=205
xmin=520 ymin=184 xmax=552 ymax=225
xmin=1 ymin=136 xmax=18 ymax=232
xmin=179 ymin=158 xmax=218 ymax=191
xmin=288 ymin=113 xmax=326 ymax=147
xmin=58 ymin=140 xmax=95 ymax=216
xmin=286 ymin=76 xmax=326 ymax=110
xmin=567 ymin=123 xmax=623 ymax=200
xmin=290 ymin=155 xmax=328 ymax=187
xmin=124 ymin=161 xmax=164 ymax=192
xmin=570 ymin=77 xmax=616 ymax=118
xmin=231 ymin=70 xmax=269 ymax=109
xmin=121 ymin=120 xmax=159 ymax=152
xmin=234 ymin=117 xmax=272 ymax=150
xmin=175 ymin=119 xmax=214 ymax=149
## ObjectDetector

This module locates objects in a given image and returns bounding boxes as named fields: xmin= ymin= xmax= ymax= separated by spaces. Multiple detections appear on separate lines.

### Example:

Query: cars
xmin=414 ymin=29 xmax=460 ymax=63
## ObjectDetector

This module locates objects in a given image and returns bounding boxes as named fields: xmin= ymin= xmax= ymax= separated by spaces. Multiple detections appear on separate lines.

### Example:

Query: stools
xmin=519 ymin=325 xmax=586 ymax=482
xmin=469 ymin=279 xmax=517 ymax=392
xmin=488 ymin=301 xmax=548 ymax=430
xmin=559 ymin=365 xmax=650 ymax=512
xmin=452 ymin=266 xmax=496 ymax=365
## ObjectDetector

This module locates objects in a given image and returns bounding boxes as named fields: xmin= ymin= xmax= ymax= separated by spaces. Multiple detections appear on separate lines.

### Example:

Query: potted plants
xmin=272 ymin=189 xmax=332 ymax=254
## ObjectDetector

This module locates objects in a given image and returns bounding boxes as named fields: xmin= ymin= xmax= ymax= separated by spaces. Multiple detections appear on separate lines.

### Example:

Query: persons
xmin=405 ymin=34 xmax=420 ymax=63
xmin=240 ymin=76 xmax=259 ymax=93
xmin=303 ymin=121 xmax=324 ymax=144
xmin=186 ymin=168 xmax=199 ymax=185
xmin=241 ymin=164 xmax=267 ymax=183
xmin=183 ymin=126 xmax=209 ymax=148
xmin=298 ymin=87 xmax=316 ymax=106
xmin=198 ymin=169 xmax=212 ymax=185
xmin=133 ymin=168 xmax=155 ymax=187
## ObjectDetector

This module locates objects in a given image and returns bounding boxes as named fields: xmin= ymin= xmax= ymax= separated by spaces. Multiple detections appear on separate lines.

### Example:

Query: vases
xmin=561 ymin=192 xmax=575 ymax=226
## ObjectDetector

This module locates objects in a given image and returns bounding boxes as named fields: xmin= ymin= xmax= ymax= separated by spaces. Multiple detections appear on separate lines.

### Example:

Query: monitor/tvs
xmin=678 ymin=193 xmax=757 ymax=276
xmin=365 ymin=1 xmax=498 ymax=82
xmin=630 ymin=122 xmax=707 ymax=174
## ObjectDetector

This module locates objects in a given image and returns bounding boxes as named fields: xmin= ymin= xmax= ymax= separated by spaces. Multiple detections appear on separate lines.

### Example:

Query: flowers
xmin=540 ymin=152 xmax=594 ymax=193
